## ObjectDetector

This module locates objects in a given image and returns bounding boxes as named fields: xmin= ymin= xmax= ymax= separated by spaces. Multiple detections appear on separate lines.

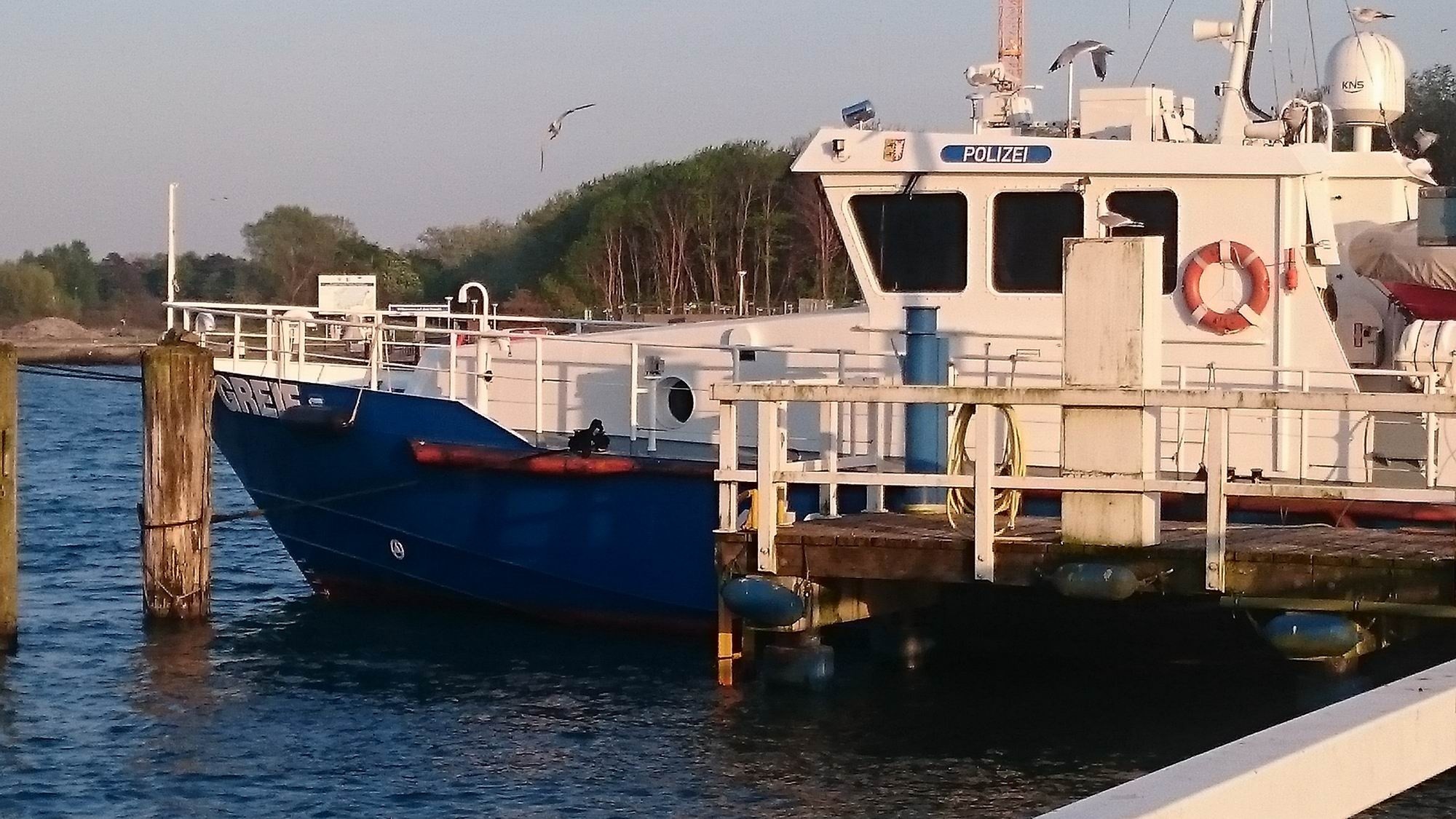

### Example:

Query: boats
xmin=1348 ymin=220 xmax=1456 ymax=321
xmin=163 ymin=0 xmax=1456 ymax=667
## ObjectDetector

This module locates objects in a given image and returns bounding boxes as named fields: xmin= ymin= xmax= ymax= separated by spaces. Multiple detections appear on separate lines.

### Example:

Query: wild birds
xmin=548 ymin=103 xmax=597 ymax=140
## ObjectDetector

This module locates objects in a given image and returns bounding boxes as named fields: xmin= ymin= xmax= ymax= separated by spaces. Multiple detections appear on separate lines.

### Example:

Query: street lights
xmin=737 ymin=270 xmax=748 ymax=317
xmin=444 ymin=297 xmax=454 ymax=329
xmin=491 ymin=304 xmax=499 ymax=330
xmin=471 ymin=300 xmax=478 ymax=321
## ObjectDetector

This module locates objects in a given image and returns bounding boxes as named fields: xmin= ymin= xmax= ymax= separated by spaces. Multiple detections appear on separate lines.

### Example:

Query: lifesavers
xmin=1182 ymin=239 xmax=1271 ymax=334
